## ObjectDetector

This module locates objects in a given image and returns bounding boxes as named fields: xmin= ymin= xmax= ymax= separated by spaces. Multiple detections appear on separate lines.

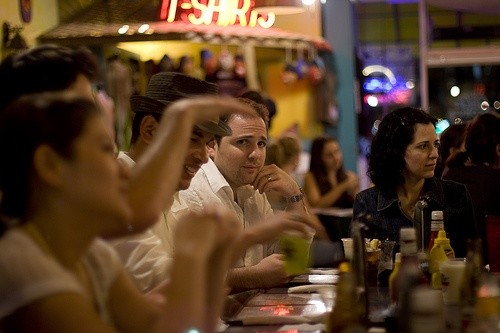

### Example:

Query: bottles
xmin=430 ymin=227 xmax=455 ymax=288
xmin=389 ymin=253 xmax=401 ymax=291
xmin=390 ymin=228 xmax=424 ymax=308
xmin=427 ymin=211 xmax=454 ymax=253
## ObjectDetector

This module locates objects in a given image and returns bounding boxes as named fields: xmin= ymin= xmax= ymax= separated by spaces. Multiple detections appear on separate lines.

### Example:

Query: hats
xmin=130 ymin=72 xmax=233 ymax=137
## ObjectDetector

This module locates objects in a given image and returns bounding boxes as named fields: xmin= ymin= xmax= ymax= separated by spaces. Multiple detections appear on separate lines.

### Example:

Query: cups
xmin=341 ymin=238 xmax=353 ymax=258
xmin=439 ymin=259 xmax=468 ymax=304
xmin=365 ymin=249 xmax=381 ymax=287
xmin=282 ymin=234 xmax=313 ymax=273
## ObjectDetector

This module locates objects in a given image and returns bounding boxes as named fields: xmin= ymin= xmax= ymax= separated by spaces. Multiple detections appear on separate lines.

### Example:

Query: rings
xmin=266 ymin=174 xmax=272 ymax=182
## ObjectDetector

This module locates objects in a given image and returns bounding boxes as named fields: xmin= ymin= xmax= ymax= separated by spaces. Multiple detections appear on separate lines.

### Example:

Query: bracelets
xmin=290 ymin=187 xmax=305 ymax=202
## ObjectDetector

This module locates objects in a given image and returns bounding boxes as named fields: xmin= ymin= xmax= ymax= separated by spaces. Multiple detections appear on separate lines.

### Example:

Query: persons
xmin=0 ymin=44 xmax=360 ymax=333
xmin=353 ymin=106 xmax=479 ymax=259
xmin=433 ymin=112 xmax=500 ymax=271
